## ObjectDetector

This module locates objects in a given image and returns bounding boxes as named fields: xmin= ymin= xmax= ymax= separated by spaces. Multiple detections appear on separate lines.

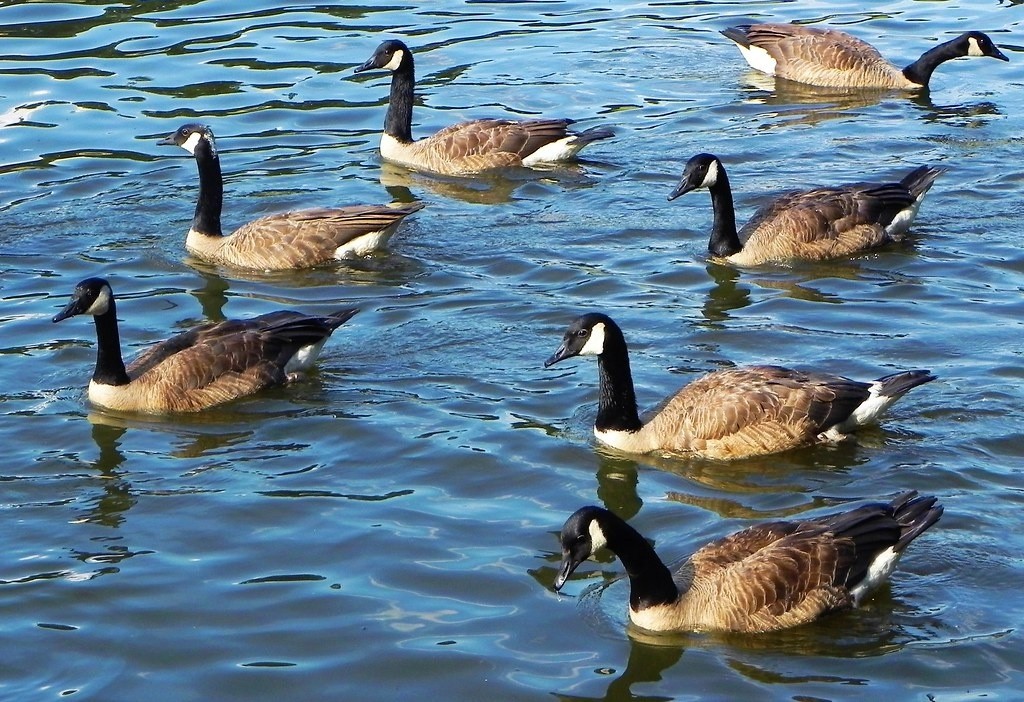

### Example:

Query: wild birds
xmin=545 ymin=312 xmax=941 ymax=462
xmin=551 ymin=487 xmax=945 ymax=635
xmin=154 ymin=122 xmax=425 ymax=272
xmin=718 ymin=23 xmax=1009 ymax=93
xmin=353 ymin=40 xmax=616 ymax=178
xmin=51 ymin=277 xmax=362 ymax=416
xmin=667 ymin=153 xmax=950 ymax=267
